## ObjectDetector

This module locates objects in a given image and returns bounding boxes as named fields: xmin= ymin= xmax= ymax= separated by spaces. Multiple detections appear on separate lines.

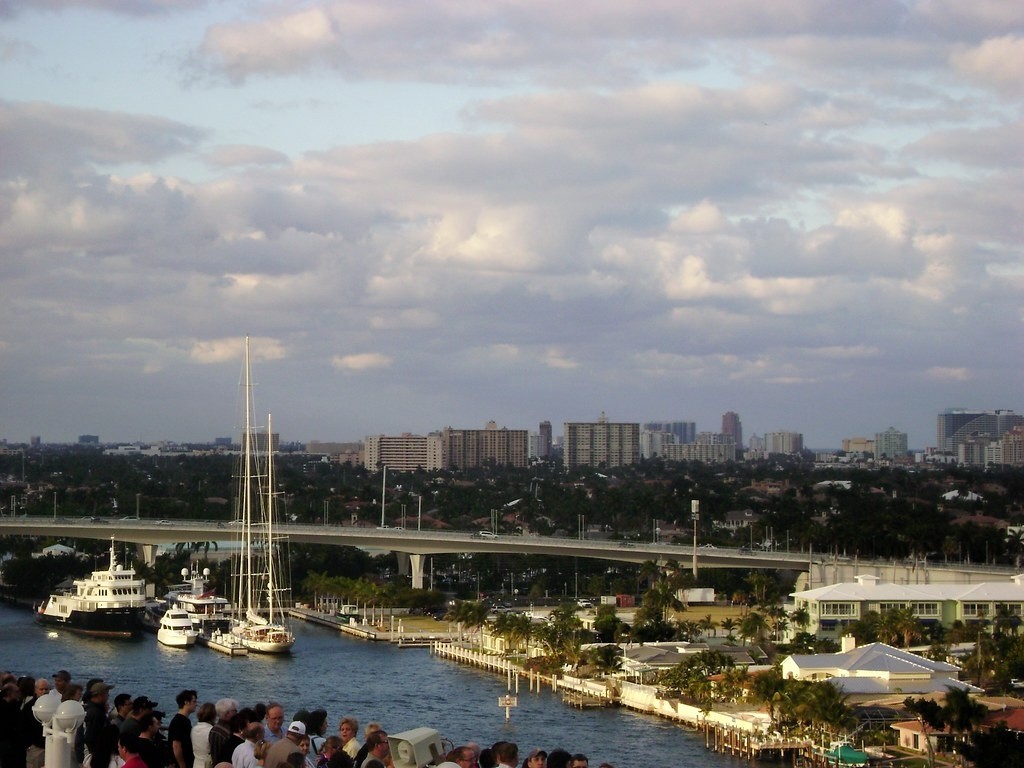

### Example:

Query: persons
xmin=440 ymin=736 xmax=520 ymax=768
xmin=168 ymin=689 xmax=198 ymax=768
xmin=117 ymin=731 xmax=148 ymax=768
xmin=361 ymin=730 xmax=390 ymax=768
xmin=86 ymin=682 xmax=115 ymax=768
xmin=354 ymin=721 xmax=395 ymax=768
xmin=547 ymin=747 xmax=572 ymax=768
xmin=21 ymin=677 xmax=50 ymax=768
xmin=522 ymin=747 xmax=548 ymax=768
xmin=570 ymin=754 xmax=588 ymax=768
xmin=264 ymin=702 xmax=289 ymax=744
xmin=317 ymin=735 xmax=355 ymax=768
xmin=49 ymin=670 xmax=71 ymax=695
xmin=0 ymin=670 xmax=328 ymax=768
xmin=599 ymin=762 xmax=614 ymax=768
xmin=231 ymin=721 xmax=265 ymax=768
xmin=338 ymin=716 xmax=361 ymax=760
xmin=264 ymin=721 xmax=306 ymax=768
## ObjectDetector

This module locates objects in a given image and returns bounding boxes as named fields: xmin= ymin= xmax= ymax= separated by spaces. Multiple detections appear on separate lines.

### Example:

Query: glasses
xmin=378 ymin=740 xmax=388 ymax=744
xmin=459 ymin=756 xmax=477 ymax=763
xmin=231 ymin=709 xmax=237 ymax=712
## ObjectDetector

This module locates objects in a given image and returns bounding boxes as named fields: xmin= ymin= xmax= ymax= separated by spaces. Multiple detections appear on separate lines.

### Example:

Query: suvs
xmin=119 ymin=516 xmax=140 ymax=521
xmin=229 ymin=519 xmax=246 ymax=526
xmin=492 ymin=606 xmax=511 ymax=614
xmin=577 ymin=599 xmax=592 ymax=608
xmin=479 ymin=531 xmax=498 ymax=538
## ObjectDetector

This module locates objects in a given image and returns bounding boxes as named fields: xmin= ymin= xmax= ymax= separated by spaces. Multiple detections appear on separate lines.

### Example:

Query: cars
xmin=493 ymin=602 xmax=511 ymax=607
xmin=378 ymin=525 xmax=391 ymax=529
xmin=158 ymin=520 xmax=175 ymax=526
xmin=699 ymin=543 xmax=717 ymax=549
xmin=80 ymin=516 xmax=100 ymax=521
xmin=392 ymin=527 xmax=404 ymax=530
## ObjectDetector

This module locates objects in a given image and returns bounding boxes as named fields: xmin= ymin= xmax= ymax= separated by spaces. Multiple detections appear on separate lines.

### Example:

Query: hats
xmin=311 ymin=709 xmax=327 ymax=719
xmin=293 ymin=708 xmax=310 ymax=721
xmin=52 ymin=670 xmax=70 ymax=680
xmin=89 ymin=682 xmax=115 ymax=695
xmin=529 ymin=749 xmax=548 ymax=758
xmin=132 ymin=696 xmax=158 ymax=707
xmin=287 ymin=721 xmax=306 ymax=735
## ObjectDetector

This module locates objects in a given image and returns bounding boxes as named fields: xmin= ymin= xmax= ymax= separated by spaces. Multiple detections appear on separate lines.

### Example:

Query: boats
xmin=36 ymin=536 xmax=148 ymax=640
xmin=157 ymin=609 xmax=198 ymax=648
xmin=147 ymin=562 xmax=237 ymax=644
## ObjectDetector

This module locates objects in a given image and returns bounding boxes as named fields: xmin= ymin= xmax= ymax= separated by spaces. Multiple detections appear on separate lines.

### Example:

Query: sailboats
xmin=200 ymin=338 xmax=252 ymax=655
xmin=230 ymin=413 xmax=296 ymax=652
